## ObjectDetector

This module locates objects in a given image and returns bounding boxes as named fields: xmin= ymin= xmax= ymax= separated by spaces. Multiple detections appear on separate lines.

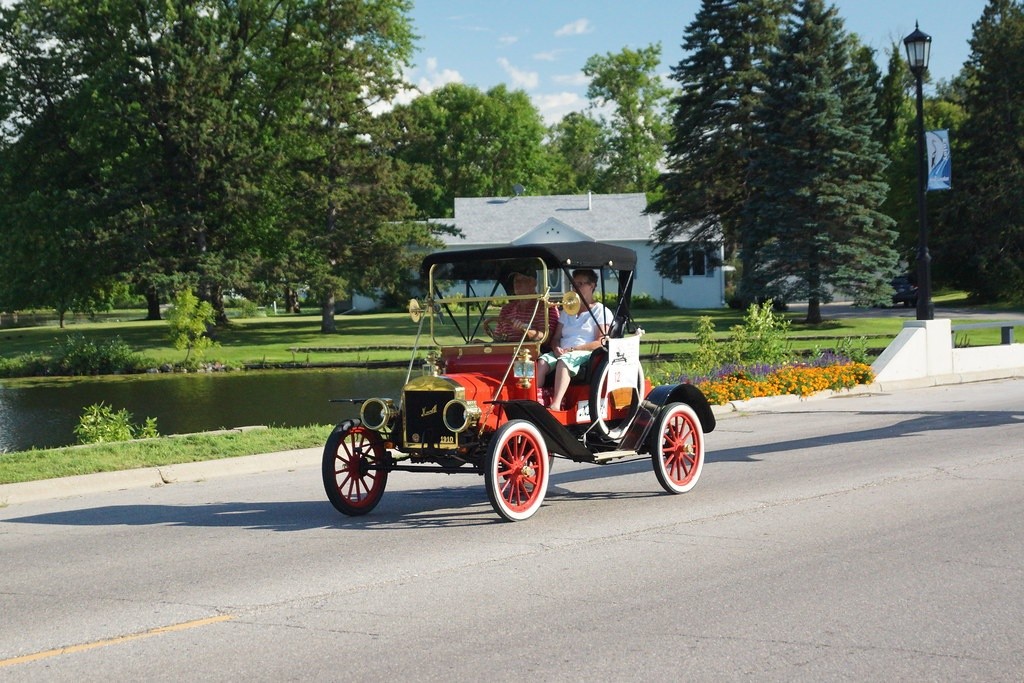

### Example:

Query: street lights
xmin=903 ymin=19 xmax=935 ymax=320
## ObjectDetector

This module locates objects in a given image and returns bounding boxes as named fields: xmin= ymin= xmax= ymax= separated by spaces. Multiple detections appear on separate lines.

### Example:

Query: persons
xmin=536 ymin=268 xmax=617 ymax=412
xmin=472 ymin=263 xmax=559 ymax=354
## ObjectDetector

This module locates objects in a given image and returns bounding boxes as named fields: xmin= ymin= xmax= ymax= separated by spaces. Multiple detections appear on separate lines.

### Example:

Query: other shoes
xmin=545 ymin=406 xmax=556 ymax=411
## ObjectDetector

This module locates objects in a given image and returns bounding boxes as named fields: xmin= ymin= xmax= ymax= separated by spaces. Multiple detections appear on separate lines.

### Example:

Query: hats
xmin=508 ymin=266 xmax=537 ymax=281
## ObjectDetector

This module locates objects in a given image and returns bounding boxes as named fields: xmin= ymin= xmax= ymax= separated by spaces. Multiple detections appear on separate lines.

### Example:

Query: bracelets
xmin=571 ymin=346 xmax=574 ymax=352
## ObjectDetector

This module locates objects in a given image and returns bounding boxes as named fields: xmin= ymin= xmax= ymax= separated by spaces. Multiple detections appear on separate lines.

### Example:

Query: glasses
xmin=569 ymin=282 xmax=589 ymax=287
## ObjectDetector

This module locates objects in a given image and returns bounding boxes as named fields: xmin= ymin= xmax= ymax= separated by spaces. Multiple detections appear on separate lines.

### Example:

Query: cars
xmin=890 ymin=277 xmax=919 ymax=307
xmin=322 ymin=241 xmax=715 ymax=521
